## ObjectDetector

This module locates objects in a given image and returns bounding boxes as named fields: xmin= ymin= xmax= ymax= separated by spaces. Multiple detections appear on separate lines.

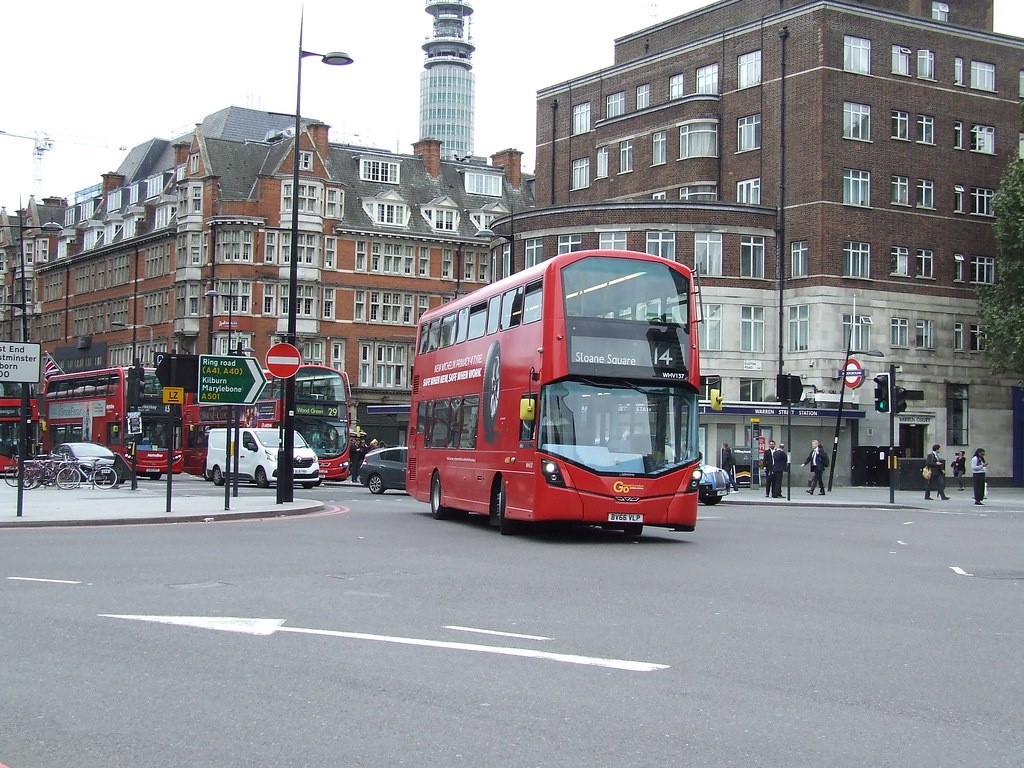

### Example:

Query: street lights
xmin=828 ymin=345 xmax=887 ymax=494
xmin=232 ymin=341 xmax=256 ymax=496
xmin=110 ymin=321 xmax=154 ymax=367
xmin=275 ymin=49 xmax=354 ymax=504
xmin=16 ymin=217 xmax=64 ymax=517
xmin=204 ymin=289 xmax=232 ymax=511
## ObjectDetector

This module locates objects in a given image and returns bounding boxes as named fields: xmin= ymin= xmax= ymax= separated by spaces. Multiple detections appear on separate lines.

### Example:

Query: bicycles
xmin=4 ymin=450 xmax=118 ymax=491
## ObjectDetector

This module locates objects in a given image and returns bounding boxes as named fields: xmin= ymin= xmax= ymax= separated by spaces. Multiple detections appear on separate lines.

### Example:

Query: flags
xmin=44 ymin=355 xmax=59 ymax=381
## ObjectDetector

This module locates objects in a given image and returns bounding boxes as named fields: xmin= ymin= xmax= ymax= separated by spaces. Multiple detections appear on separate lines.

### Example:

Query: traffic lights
xmin=873 ymin=371 xmax=892 ymax=415
xmin=895 ymin=386 xmax=907 ymax=413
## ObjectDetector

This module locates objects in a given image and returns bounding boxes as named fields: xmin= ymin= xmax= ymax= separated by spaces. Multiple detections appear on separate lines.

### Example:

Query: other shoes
xmin=975 ymin=502 xmax=985 ymax=506
xmin=819 ymin=493 xmax=825 ymax=495
xmin=766 ymin=494 xmax=768 ymax=497
xmin=925 ymin=498 xmax=933 ymax=500
xmin=942 ymin=497 xmax=949 ymax=500
xmin=806 ymin=490 xmax=813 ymax=495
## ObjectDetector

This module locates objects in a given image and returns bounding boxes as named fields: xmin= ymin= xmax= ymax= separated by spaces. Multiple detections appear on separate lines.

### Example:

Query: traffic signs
xmin=196 ymin=353 xmax=267 ymax=407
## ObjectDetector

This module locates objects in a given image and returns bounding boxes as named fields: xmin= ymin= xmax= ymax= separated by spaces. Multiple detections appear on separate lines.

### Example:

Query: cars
xmin=49 ymin=441 xmax=115 ymax=481
xmin=358 ymin=443 xmax=406 ymax=494
xmin=699 ymin=463 xmax=731 ymax=505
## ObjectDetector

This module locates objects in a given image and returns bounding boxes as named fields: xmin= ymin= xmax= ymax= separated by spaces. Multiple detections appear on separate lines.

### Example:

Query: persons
xmin=763 ymin=441 xmax=777 ymax=497
xmin=350 ymin=437 xmax=384 ymax=482
xmin=925 ymin=445 xmax=950 ymax=500
xmin=772 ymin=444 xmax=787 ymax=498
xmin=951 ymin=451 xmax=966 ymax=491
xmin=722 ymin=443 xmax=739 ymax=493
xmin=801 ymin=440 xmax=825 ymax=495
xmin=970 ymin=448 xmax=988 ymax=506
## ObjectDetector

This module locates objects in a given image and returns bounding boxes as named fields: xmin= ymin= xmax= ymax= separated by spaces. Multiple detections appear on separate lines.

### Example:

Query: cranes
xmin=0 ymin=128 xmax=128 ymax=204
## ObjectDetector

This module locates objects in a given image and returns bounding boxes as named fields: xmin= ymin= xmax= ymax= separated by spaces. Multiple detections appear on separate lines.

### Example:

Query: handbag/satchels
xmin=922 ymin=466 xmax=932 ymax=479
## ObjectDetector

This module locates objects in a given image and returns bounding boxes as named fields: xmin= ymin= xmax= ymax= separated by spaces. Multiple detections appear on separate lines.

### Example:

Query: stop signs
xmin=264 ymin=342 xmax=301 ymax=378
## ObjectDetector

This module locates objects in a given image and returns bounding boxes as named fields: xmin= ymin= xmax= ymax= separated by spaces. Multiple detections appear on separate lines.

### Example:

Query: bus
xmin=183 ymin=365 xmax=361 ymax=482
xmin=0 ymin=381 xmax=41 ymax=478
xmin=406 ymin=249 xmax=724 ymax=539
xmin=42 ymin=366 xmax=184 ymax=481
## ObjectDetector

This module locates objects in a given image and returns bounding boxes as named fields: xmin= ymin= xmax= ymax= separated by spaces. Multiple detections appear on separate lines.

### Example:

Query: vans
xmin=207 ymin=427 xmax=320 ymax=490
